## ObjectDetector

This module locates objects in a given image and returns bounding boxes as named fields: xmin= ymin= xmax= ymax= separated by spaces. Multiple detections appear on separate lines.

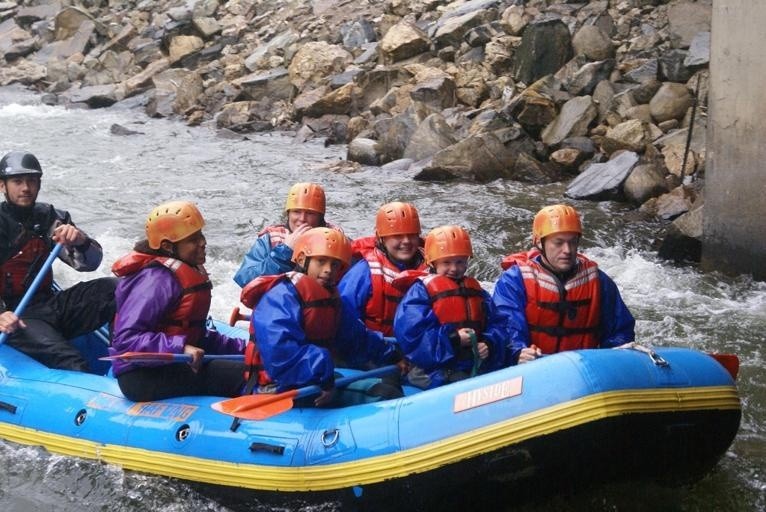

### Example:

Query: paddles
xmin=97 ymin=351 xmax=246 ymax=366
xmin=710 ymin=353 xmax=739 ymax=381
xmin=211 ymin=364 xmax=401 ymax=421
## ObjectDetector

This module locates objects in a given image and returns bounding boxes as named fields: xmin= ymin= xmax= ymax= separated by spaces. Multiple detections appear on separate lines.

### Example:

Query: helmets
xmin=0 ymin=151 xmax=42 ymax=178
xmin=146 ymin=201 xmax=205 ymax=250
xmin=285 ymin=183 xmax=326 ymax=215
xmin=291 ymin=226 xmax=351 ymax=265
xmin=534 ymin=205 xmax=583 ymax=242
xmin=424 ymin=225 xmax=474 ymax=265
xmin=376 ymin=202 xmax=422 ymax=237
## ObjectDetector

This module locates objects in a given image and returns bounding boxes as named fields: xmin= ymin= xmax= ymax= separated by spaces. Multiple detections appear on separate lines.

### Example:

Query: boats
xmin=0 ymin=296 xmax=747 ymax=504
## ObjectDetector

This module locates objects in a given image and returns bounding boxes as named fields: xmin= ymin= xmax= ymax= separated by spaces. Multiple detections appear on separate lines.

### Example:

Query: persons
xmin=335 ymin=200 xmax=437 ymax=368
xmin=391 ymin=222 xmax=498 ymax=392
xmin=106 ymin=201 xmax=251 ymax=402
xmin=0 ymin=148 xmax=119 ymax=372
xmin=491 ymin=204 xmax=637 ymax=366
xmin=231 ymin=181 xmax=363 ymax=290
xmin=241 ymin=226 xmax=406 ymax=409
